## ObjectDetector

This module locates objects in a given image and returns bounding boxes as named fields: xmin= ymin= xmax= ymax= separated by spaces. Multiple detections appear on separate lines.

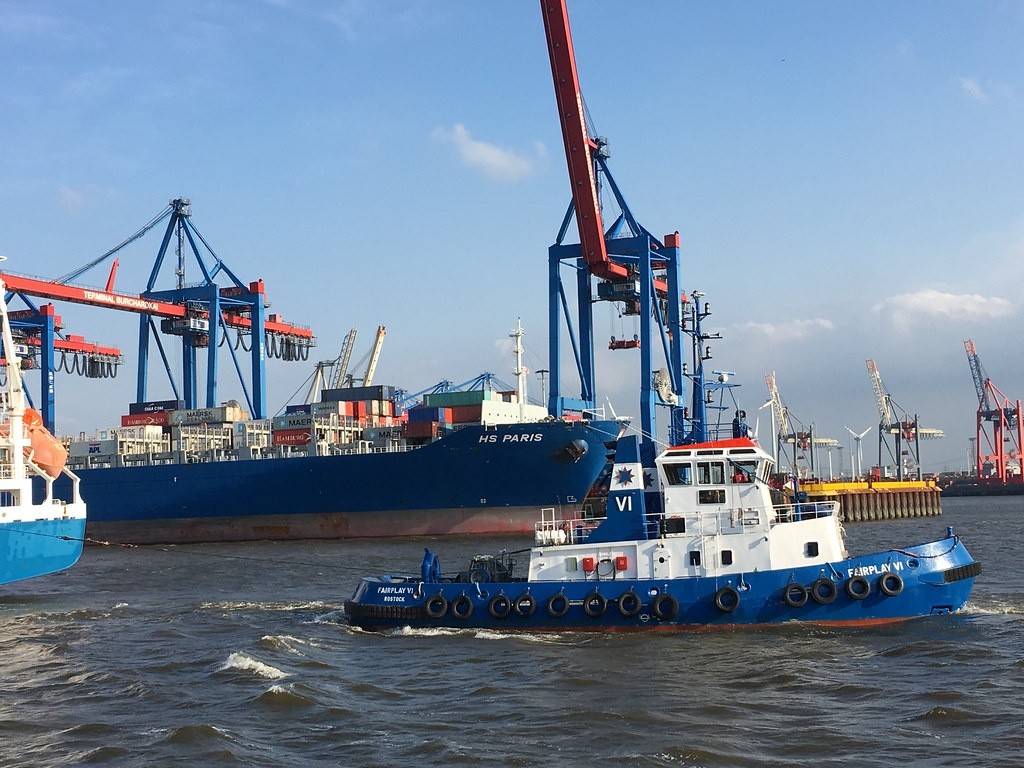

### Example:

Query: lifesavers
xmin=469 ymin=569 xmax=491 ymax=585
xmin=425 ymin=595 xmax=448 ymax=618
xmin=452 ymin=596 xmax=473 ymax=619
xmin=715 ymin=587 xmax=739 ymax=612
xmin=846 ymin=576 xmax=870 ymax=600
xmin=618 ymin=591 xmax=642 ymax=616
xmin=488 ymin=594 xmax=510 ymax=617
xmin=811 ymin=578 xmax=837 ymax=604
xmin=784 ymin=583 xmax=808 ymax=607
xmin=547 ymin=594 xmax=569 ymax=617
xmin=514 ymin=594 xmax=536 ymax=617
xmin=654 ymin=594 xmax=678 ymax=618
xmin=880 ymin=573 xmax=904 ymax=596
xmin=584 ymin=593 xmax=606 ymax=616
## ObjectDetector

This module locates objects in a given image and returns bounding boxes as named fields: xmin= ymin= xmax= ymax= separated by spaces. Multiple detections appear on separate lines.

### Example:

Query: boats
xmin=1 ymin=253 xmax=88 ymax=585
xmin=30 ymin=314 xmax=982 ymax=637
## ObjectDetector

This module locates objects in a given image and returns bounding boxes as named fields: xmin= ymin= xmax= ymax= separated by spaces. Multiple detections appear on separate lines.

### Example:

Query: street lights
xmin=534 ymin=369 xmax=550 ymax=407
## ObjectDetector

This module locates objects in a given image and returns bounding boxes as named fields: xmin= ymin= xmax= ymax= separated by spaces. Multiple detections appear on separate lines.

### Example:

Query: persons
xmin=733 ymin=470 xmax=748 ymax=483
